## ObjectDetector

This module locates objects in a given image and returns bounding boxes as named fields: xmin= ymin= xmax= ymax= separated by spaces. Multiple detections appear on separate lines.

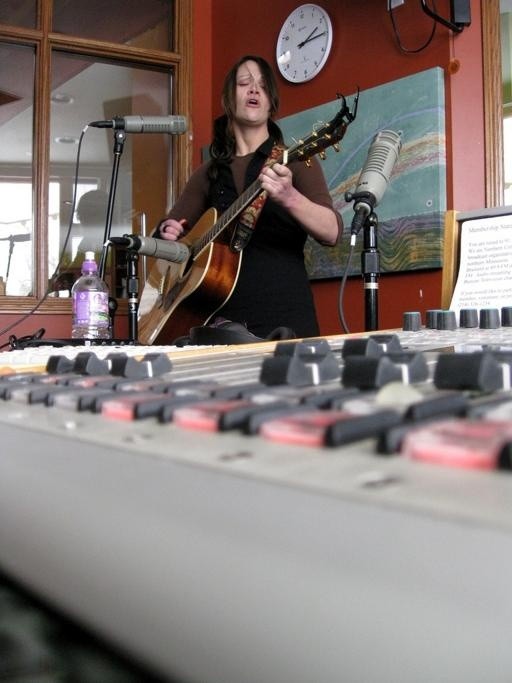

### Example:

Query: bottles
xmin=72 ymin=249 xmax=112 ymax=338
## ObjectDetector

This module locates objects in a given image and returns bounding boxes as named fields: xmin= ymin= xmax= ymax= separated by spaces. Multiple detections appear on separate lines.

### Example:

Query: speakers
xmin=450 ymin=0 xmax=471 ymax=27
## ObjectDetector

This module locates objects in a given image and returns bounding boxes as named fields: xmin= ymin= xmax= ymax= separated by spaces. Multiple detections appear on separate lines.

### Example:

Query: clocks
xmin=276 ymin=2 xmax=334 ymax=83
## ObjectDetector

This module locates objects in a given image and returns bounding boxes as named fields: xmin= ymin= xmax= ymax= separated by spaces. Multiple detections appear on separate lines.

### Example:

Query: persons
xmin=149 ymin=53 xmax=344 ymax=339
xmin=54 ymin=189 xmax=123 ymax=292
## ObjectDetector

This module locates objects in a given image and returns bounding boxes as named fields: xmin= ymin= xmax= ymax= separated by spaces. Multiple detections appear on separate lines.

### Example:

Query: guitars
xmin=137 ymin=85 xmax=359 ymax=344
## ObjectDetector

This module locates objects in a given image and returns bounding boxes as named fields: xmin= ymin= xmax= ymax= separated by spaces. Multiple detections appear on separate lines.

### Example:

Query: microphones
xmin=126 ymin=235 xmax=189 ymax=265
xmin=114 ymin=115 xmax=187 ymax=134
xmin=352 ymin=128 xmax=402 ymax=214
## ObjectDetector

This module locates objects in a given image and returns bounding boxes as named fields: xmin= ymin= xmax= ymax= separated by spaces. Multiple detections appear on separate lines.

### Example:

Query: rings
xmin=159 ymin=223 xmax=170 ymax=231
